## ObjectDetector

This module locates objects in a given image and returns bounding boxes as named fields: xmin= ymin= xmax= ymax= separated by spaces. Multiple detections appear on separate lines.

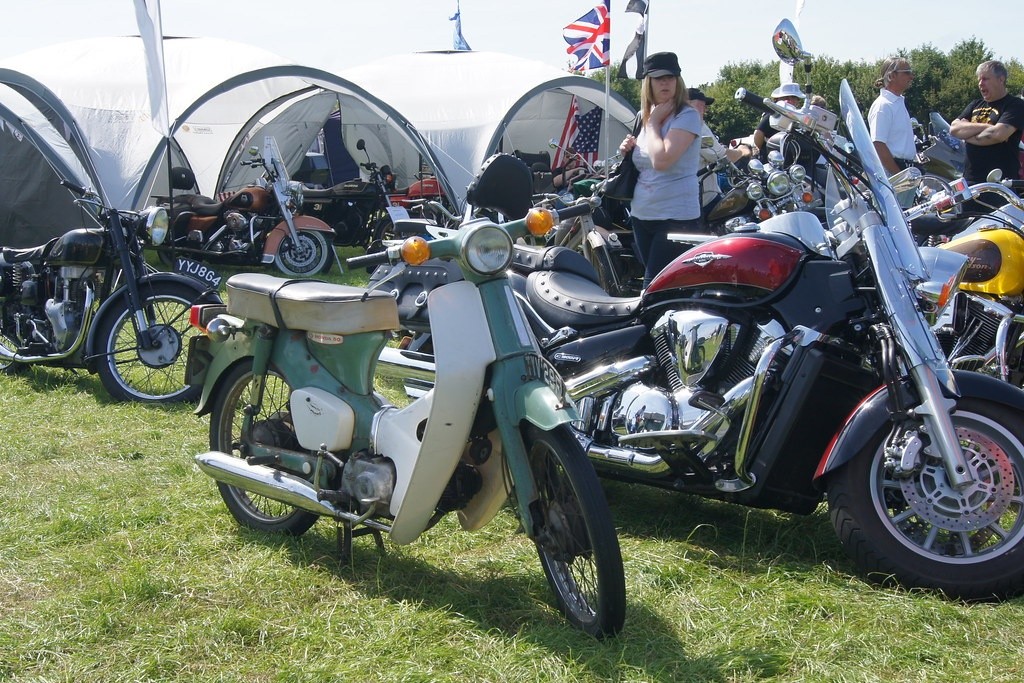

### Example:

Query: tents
xmin=0 ymin=1 xmax=670 ymax=297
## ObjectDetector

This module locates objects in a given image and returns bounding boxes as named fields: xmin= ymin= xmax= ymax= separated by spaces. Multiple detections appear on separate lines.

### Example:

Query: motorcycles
xmin=2 ymin=17 xmax=1024 ymax=641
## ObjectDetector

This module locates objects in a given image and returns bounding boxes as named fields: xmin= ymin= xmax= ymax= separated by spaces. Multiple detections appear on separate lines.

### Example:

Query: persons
xmin=617 ymin=52 xmax=703 ymax=386
xmin=948 ymin=61 xmax=1024 ymax=214
xmin=809 ymin=94 xmax=849 ymax=202
xmin=681 ymin=87 xmax=752 ymax=222
xmin=868 ymin=56 xmax=925 ymax=208
xmin=754 ymin=81 xmax=806 ymax=208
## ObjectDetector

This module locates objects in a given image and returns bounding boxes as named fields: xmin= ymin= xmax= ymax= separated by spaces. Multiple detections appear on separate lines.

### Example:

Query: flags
xmin=129 ymin=0 xmax=166 ymax=121
xmin=560 ymin=0 xmax=609 ymax=72
xmin=616 ymin=0 xmax=648 ymax=79
xmin=551 ymin=92 xmax=603 ymax=178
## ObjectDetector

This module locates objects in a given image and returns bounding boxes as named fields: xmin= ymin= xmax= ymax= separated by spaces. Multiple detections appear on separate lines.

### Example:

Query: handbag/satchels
xmin=605 ymin=147 xmax=640 ymax=199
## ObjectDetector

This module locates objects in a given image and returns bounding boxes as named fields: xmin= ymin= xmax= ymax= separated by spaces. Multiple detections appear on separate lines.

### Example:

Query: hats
xmin=771 ymin=83 xmax=806 ymax=99
xmin=643 ymin=52 xmax=681 ymax=78
xmin=686 ymin=88 xmax=714 ymax=105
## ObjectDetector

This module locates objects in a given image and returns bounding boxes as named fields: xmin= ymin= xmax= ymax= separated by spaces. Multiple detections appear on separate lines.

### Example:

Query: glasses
xmin=894 ymin=69 xmax=912 ymax=73
